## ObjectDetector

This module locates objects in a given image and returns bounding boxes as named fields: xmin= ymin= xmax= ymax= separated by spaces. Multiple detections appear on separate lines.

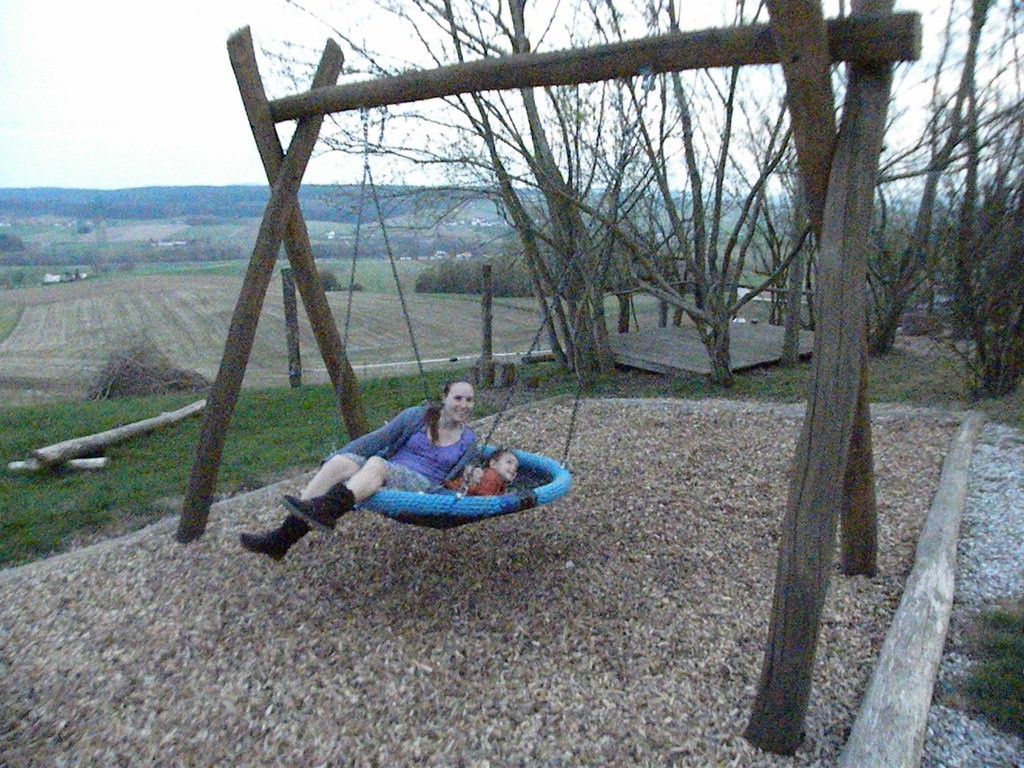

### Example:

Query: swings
xmin=323 ymin=66 xmax=659 ymax=533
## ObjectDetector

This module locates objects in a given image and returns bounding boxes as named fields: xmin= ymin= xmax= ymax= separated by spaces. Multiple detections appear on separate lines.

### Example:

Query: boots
xmin=280 ymin=482 xmax=355 ymax=532
xmin=239 ymin=513 xmax=313 ymax=562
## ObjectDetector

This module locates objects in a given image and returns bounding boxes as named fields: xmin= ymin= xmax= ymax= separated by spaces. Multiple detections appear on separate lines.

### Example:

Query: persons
xmin=445 ymin=449 xmax=519 ymax=496
xmin=239 ymin=379 xmax=476 ymax=560
